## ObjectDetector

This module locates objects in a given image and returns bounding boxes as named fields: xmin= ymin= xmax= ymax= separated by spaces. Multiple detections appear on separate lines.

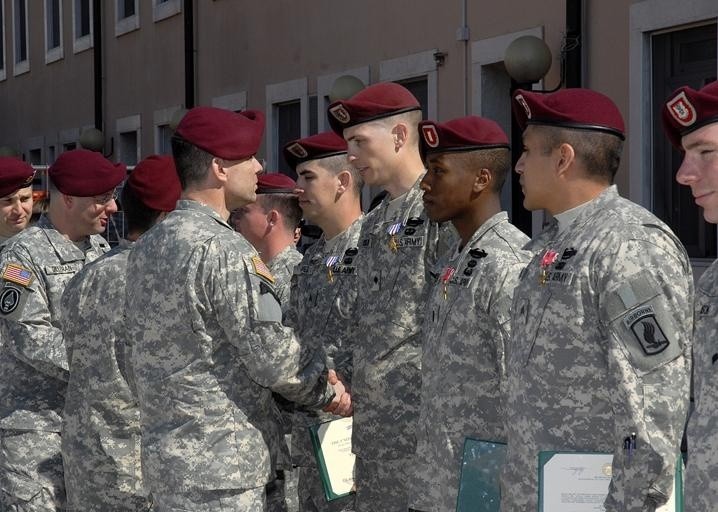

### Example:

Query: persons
xmin=409 ymin=117 xmax=530 ymax=511
xmin=506 ymin=87 xmax=693 ymax=512
xmin=2 ymin=146 xmax=114 ymax=512
xmin=663 ymin=80 xmax=716 ymax=510
xmin=122 ymin=107 xmax=345 ymax=511
xmin=61 ymin=154 xmax=177 ymax=510
xmin=288 ymin=131 xmax=358 ymax=511
xmin=231 ymin=172 xmax=312 ymax=295
xmin=326 ymin=82 xmax=416 ymax=511
xmin=0 ymin=153 xmax=35 ymax=239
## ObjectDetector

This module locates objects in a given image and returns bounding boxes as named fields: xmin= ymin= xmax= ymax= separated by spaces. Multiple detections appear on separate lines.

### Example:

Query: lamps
xmin=80 ymin=127 xmax=114 ymax=158
xmin=504 ymin=36 xmax=564 ymax=97
xmin=1 ymin=146 xmax=18 ymax=157
xmin=169 ymin=109 xmax=190 ymax=131
xmin=329 ymin=74 xmax=365 ymax=102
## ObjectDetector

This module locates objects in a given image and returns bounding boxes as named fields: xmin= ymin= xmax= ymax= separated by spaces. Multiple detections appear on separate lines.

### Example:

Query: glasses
xmin=100 ymin=191 xmax=118 ymax=202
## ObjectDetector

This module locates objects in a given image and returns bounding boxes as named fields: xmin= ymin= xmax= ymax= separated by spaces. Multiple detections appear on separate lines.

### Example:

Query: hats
xmin=175 ymin=105 xmax=265 ymax=161
xmin=417 ymin=115 xmax=512 ymax=163
xmin=256 ymin=172 xmax=296 ymax=192
xmin=47 ymin=149 xmax=127 ymax=197
xmin=664 ymin=80 xmax=718 ymax=136
xmin=0 ymin=155 xmax=37 ymax=199
xmin=513 ymin=87 xmax=626 ymax=141
xmin=326 ymin=82 xmax=421 ymax=139
xmin=128 ymin=153 xmax=182 ymax=212
xmin=283 ymin=130 xmax=349 ymax=167
